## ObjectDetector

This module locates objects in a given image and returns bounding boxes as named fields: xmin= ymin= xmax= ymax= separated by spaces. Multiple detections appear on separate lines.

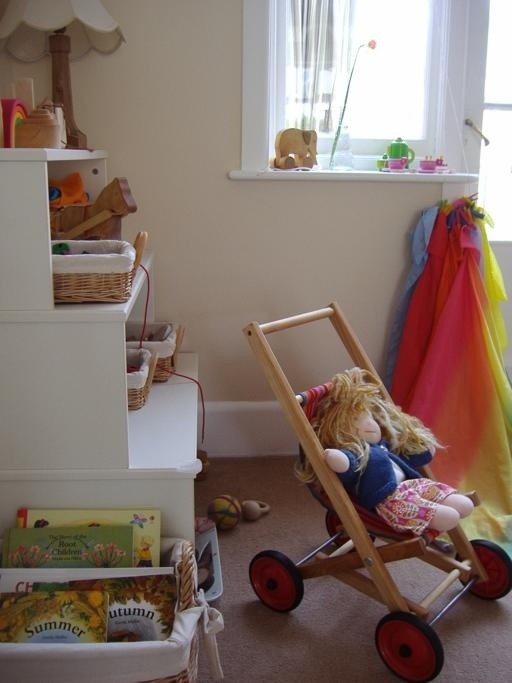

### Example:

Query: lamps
xmin=0 ymin=1 xmax=129 ymax=149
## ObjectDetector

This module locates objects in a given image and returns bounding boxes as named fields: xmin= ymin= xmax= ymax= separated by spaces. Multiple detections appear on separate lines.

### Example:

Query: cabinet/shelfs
xmin=1 ymin=147 xmax=199 ymax=553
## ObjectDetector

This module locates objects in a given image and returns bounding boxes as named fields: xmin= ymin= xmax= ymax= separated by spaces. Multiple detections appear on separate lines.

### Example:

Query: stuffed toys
xmin=293 ymin=367 xmax=474 ymax=536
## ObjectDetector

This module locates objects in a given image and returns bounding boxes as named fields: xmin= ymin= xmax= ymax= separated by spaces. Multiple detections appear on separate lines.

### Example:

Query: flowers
xmin=330 ymin=40 xmax=375 ymax=164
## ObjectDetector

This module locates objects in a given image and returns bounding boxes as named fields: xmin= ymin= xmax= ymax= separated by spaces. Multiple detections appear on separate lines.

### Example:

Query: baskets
xmin=126 ymin=321 xmax=176 ymax=409
xmin=51 ymin=240 xmax=136 ymax=303
xmin=0 ymin=537 xmax=199 ymax=683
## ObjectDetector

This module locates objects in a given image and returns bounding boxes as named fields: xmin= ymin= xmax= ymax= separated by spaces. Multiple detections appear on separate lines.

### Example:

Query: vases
xmin=330 ymin=114 xmax=352 ymax=171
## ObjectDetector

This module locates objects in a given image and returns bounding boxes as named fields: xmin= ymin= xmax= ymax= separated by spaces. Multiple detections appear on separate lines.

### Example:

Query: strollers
xmin=237 ymin=300 xmax=512 ymax=682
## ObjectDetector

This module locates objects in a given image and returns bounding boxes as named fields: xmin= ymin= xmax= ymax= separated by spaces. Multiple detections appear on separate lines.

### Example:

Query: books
xmin=0 ymin=508 xmax=177 ymax=644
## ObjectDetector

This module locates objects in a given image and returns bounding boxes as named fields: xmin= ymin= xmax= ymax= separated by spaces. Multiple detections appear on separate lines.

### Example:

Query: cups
xmin=377 ymin=153 xmax=449 ymax=175
xmin=14 ymin=74 xmax=35 ymax=111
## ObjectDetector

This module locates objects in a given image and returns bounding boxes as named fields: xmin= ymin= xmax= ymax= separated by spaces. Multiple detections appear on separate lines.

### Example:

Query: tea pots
xmin=386 ymin=135 xmax=416 ymax=170
